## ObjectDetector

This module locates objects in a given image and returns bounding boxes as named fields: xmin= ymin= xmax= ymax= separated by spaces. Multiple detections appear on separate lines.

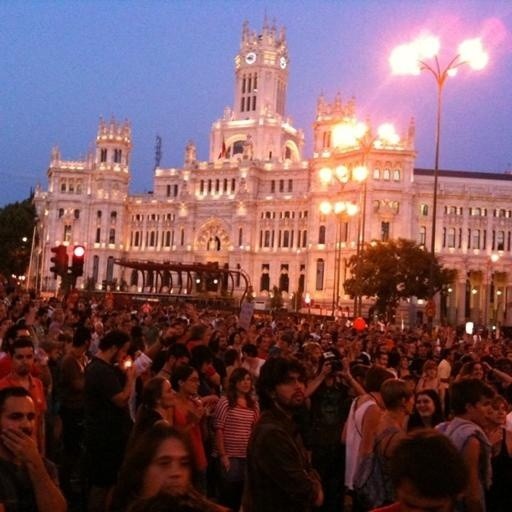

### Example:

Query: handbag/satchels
xmin=129 ymin=429 xmax=162 ymax=468
xmin=352 ymin=428 xmax=396 ymax=507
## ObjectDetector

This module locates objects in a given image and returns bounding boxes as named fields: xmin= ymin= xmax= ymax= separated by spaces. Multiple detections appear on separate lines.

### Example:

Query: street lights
xmin=73 ymin=245 xmax=85 ymax=277
xmin=482 ymin=251 xmax=501 ymax=323
xmin=390 ymin=33 xmax=487 ymax=335
xmin=330 ymin=116 xmax=399 ymax=317
xmin=22 ymin=209 xmax=49 ymax=292
xmin=317 ymin=162 xmax=368 ymax=316
xmin=49 ymin=246 xmax=59 ymax=273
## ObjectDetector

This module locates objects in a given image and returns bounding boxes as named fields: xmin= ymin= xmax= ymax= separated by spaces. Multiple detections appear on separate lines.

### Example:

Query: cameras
xmin=318 ymin=351 xmax=343 ymax=374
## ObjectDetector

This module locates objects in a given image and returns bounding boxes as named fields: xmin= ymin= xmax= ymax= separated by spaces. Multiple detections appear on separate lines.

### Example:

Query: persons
xmin=1 ymin=276 xmax=511 ymax=512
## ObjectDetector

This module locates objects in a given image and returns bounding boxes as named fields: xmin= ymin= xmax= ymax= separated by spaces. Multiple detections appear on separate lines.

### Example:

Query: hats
xmin=363 ymin=366 xmax=396 ymax=392
